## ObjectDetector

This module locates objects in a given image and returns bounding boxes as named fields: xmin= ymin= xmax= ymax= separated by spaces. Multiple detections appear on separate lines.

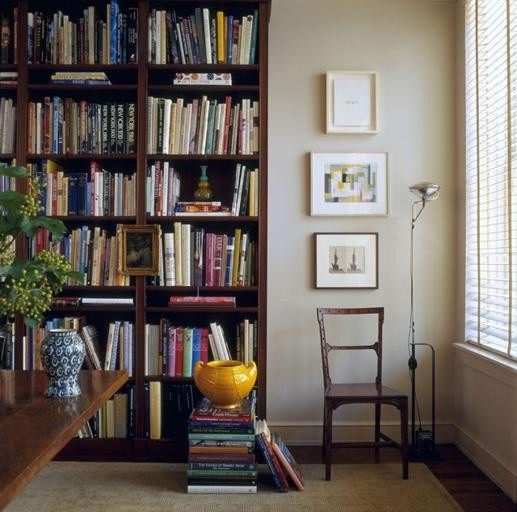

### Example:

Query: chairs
xmin=314 ymin=308 xmax=409 ymax=483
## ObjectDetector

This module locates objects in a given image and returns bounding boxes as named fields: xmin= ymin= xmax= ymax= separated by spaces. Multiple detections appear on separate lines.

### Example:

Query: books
xmin=0 ymin=321 xmax=15 ymax=371
xmin=145 ymin=95 xmax=259 ymax=155
xmin=167 ymin=295 xmax=236 ymax=307
xmin=26 ymin=1 xmax=138 ymax=64
xmin=254 ymin=418 xmax=305 ymax=494
xmin=147 ymin=6 xmax=258 ymax=63
xmin=142 ymin=380 xmax=193 ymax=441
xmin=50 ymin=72 xmax=112 ymax=86
xmin=171 ymin=71 xmax=232 ymax=85
xmin=26 ymin=95 xmax=136 ymax=155
xmin=29 ymin=225 xmax=130 ymax=286
xmin=27 ymin=159 xmax=137 ymax=215
xmin=149 ymin=222 xmax=255 ymax=287
xmin=185 ymin=388 xmax=258 ymax=495
xmin=22 ymin=312 xmax=133 ymax=378
xmin=0 ymin=2 xmax=18 ymax=65
xmin=146 ymin=159 xmax=259 ymax=218
xmin=0 ymin=95 xmax=16 ymax=153
xmin=144 ymin=318 xmax=258 ymax=377
xmin=72 ymin=385 xmax=134 ymax=439
xmin=0 ymin=70 xmax=18 ymax=84
xmin=0 ymin=158 xmax=17 ymax=215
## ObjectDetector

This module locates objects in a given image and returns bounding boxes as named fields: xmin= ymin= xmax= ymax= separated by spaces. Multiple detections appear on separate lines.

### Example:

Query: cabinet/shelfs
xmin=0 ymin=0 xmax=271 ymax=462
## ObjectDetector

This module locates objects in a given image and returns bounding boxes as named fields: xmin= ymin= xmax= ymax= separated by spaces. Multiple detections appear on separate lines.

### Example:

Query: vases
xmin=41 ymin=330 xmax=84 ymax=397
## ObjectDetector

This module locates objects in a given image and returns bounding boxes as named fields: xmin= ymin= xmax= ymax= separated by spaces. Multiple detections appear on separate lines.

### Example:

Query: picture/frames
xmin=307 ymin=149 xmax=391 ymax=220
xmin=310 ymin=230 xmax=380 ymax=292
xmin=323 ymin=68 xmax=380 ymax=133
xmin=116 ymin=223 xmax=161 ymax=278
